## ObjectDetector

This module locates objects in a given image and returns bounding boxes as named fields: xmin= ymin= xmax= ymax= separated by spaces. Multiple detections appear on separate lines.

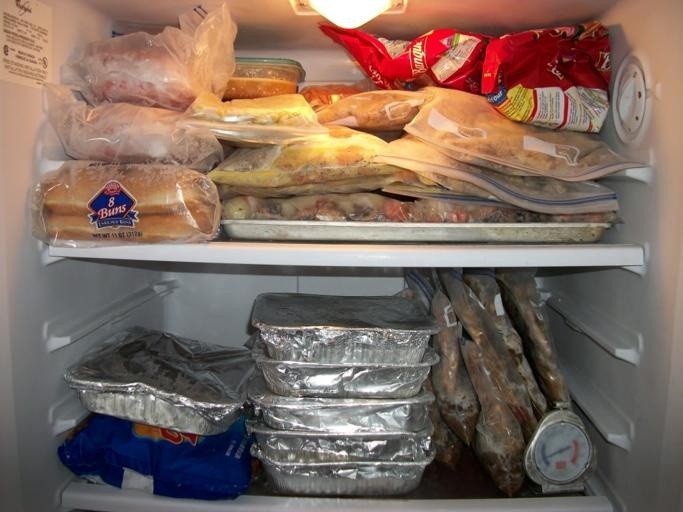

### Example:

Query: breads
xmin=42 ymin=164 xmax=218 ymax=243
